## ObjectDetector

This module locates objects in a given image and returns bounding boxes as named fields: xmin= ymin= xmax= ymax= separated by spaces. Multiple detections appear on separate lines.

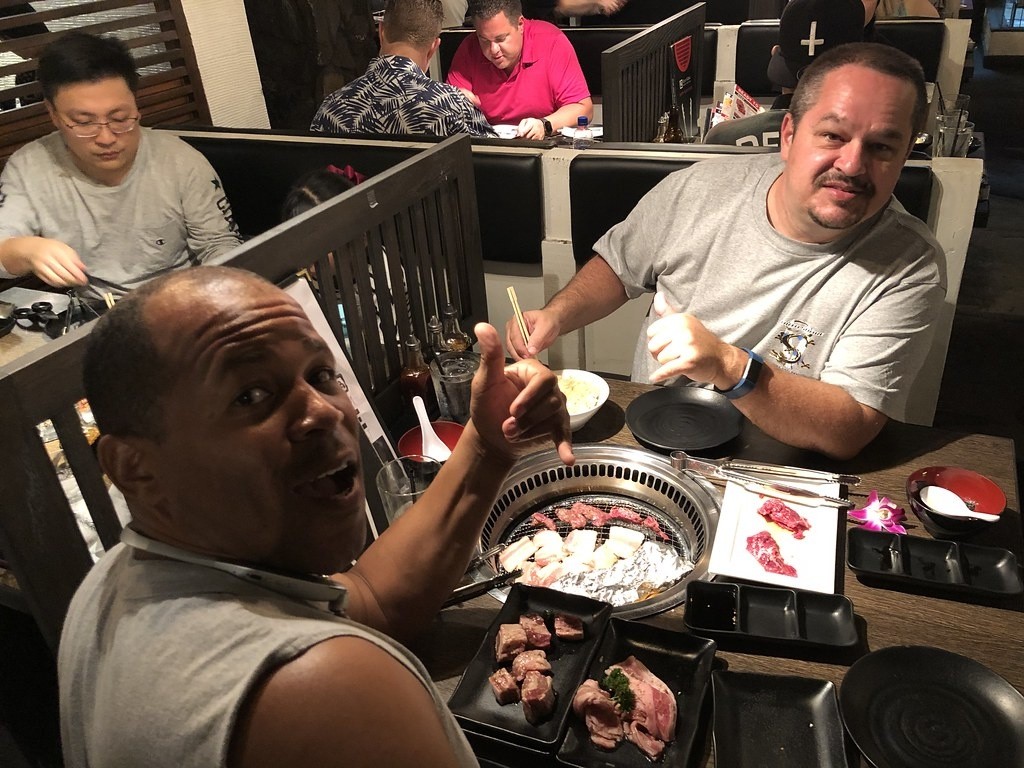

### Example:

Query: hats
xmin=769 ymin=0 xmax=868 ymax=89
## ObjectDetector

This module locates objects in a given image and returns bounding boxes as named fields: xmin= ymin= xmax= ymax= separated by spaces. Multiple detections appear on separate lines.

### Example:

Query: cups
xmin=430 ymin=349 xmax=481 ymax=427
xmin=376 ymin=455 xmax=443 ymax=527
xmin=936 ymin=93 xmax=975 ymax=158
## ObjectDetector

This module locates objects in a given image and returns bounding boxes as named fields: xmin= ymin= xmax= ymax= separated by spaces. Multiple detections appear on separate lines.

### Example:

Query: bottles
xmin=651 ymin=106 xmax=684 ymax=144
xmin=401 ymin=303 xmax=473 ymax=421
xmin=573 ymin=116 xmax=593 ymax=149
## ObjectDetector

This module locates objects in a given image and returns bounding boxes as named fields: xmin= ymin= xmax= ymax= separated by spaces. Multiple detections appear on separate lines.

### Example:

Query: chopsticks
xmin=104 ymin=293 xmax=115 ymax=309
xmin=507 ymin=286 xmax=538 ymax=361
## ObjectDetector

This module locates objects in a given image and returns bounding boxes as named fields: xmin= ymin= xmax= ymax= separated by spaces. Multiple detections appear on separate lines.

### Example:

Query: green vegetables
xmin=602 ymin=668 xmax=637 ymax=714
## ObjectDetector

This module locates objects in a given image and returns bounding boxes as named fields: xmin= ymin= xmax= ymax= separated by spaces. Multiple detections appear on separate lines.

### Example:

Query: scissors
xmin=10 ymin=301 xmax=66 ymax=319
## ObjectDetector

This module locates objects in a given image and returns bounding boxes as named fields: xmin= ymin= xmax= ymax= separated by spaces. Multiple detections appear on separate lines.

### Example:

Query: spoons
xmin=412 ymin=396 xmax=451 ymax=463
xmin=920 ymin=486 xmax=1000 ymax=523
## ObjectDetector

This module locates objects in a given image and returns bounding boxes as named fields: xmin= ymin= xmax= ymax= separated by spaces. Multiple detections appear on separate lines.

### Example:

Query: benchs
xmin=151 ymin=3 xmax=984 ymax=436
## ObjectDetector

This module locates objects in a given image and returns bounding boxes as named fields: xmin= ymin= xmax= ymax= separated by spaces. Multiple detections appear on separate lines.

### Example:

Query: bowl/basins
xmin=551 ymin=369 xmax=610 ymax=432
xmin=907 ymin=132 xmax=982 ymax=160
xmin=906 ymin=466 xmax=1007 ymax=540
xmin=0 ymin=301 xmax=17 ymax=337
xmin=45 ymin=299 xmax=120 ymax=339
xmin=398 ymin=421 xmax=465 ymax=466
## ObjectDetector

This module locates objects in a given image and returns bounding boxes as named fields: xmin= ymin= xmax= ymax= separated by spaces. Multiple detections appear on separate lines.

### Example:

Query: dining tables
xmin=412 ymin=376 xmax=1024 ymax=768
xmin=0 ymin=286 xmax=133 ymax=614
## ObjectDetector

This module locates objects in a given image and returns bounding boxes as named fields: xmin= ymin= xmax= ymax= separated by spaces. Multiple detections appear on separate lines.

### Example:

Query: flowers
xmin=847 ymin=489 xmax=908 ymax=537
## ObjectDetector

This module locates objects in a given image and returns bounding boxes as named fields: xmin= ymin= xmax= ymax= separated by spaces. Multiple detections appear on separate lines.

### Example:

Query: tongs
xmin=670 ymin=451 xmax=861 ymax=508
xmin=65 ymin=289 xmax=101 ymax=333
xmin=440 ymin=544 xmax=524 ymax=610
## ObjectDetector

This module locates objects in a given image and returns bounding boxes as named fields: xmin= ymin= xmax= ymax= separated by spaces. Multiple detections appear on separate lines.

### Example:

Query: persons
xmin=285 ymin=165 xmax=419 ymax=380
xmin=441 ymin=0 xmax=628 ymax=27
xmin=311 ymin=0 xmax=499 ymax=138
xmin=771 ymin=0 xmax=941 ymax=113
xmin=445 ymin=0 xmax=593 ymax=141
xmin=59 ymin=266 xmax=576 ymax=768
xmin=506 ymin=41 xmax=948 ymax=457
xmin=0 ymin=34 xmax=243 ymax=301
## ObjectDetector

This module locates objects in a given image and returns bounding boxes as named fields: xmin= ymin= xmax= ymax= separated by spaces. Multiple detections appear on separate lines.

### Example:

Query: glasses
xmin=51 ymin=99 xmax=140 ymax=139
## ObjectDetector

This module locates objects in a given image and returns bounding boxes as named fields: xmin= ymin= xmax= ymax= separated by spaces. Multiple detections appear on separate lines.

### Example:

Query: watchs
xmin=714 ymin=347 xmax=763 ymax=398
xmin=540 ymin=117 xmax=552 ymax=136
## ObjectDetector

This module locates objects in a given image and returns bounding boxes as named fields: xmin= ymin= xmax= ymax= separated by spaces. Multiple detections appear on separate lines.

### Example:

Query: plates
xmin=683 ymin=460 xmax=1023 ymax=645
xmin=487 ymin=124 xmax=603 ymax=139
xmin=839 ymin=645 xmax=1024 ymax=768
xmin=625 ymin=386 xmax=744 ymax=450
xmin=712 ymin=670 xmax=848 ymax=768
xmin=445 ymin=582 xmax=716 ymax=768
